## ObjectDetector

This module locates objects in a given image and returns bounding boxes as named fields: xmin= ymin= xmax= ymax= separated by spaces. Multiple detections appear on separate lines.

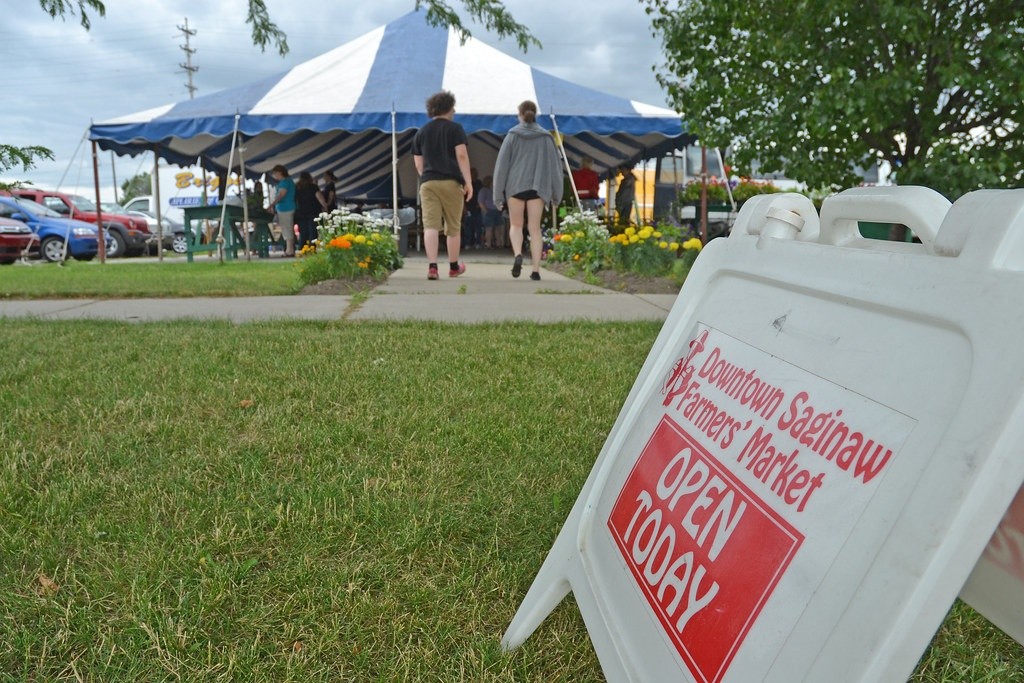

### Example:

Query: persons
xmin=321 ymin=171 xmax=338 ymax=219
xmin=492 ymin=100 xmax=564 ymax=281
xmin=462 ymin=168 xmax=509 ymax=250
xmin=615 ymin=164 xmax=637 ymax=224
xmin=410 ymin=92 xmax=474 ymax=279
xmin=352 ymin=202 xmax=364 ymax=215
xmin=266 ymin=165 xmax=297 ymax=258
xmin=294 ymin=172 xmax=327 ymax=251
xmin=571 ymin=157 xmax=600 ymax=211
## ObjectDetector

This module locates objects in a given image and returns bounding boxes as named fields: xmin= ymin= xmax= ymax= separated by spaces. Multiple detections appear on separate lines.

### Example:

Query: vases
xmin=684 ymin=201 xmax=723 ymax=206
xmin=726 ymin=201 xmax=731 ymax=205
xmin=737 ymin=201 xmax=742 ymax=205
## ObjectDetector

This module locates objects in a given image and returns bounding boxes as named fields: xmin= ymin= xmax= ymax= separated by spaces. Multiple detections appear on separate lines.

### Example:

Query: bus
xmin=599 ymin=121 xmax=907 ymax=231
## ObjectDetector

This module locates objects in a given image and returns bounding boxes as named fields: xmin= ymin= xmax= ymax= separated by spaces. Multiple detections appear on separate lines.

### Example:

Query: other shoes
xmin=282 ymin=252 xmax=294 ymax=257
xmin=531 ymin=272 xmax=540 ymax=280
xmin=512 ymin=255 xmax=522 ymax=277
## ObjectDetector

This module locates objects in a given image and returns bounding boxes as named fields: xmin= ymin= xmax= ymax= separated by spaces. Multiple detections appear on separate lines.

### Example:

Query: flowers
xmin=681 ymin=177 xmax=779 ymax=202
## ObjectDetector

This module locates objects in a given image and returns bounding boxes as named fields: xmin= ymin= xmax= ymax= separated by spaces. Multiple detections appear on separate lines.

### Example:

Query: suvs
xmin=10 ymin=189 xmax=149 ymax=258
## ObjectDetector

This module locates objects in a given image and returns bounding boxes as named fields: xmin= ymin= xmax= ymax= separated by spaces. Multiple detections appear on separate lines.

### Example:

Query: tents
xmin=88 ymin=5 xmax=708 ymax=267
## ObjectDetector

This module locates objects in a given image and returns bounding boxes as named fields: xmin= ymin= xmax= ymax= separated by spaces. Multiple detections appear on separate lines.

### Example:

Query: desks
xmin=183 ymin=205 xmax=275 ymax=262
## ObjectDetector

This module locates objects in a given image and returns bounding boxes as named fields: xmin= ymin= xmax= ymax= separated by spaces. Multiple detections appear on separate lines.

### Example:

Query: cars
xmin=118 ymin=210 xmax=173 ymax=246
xmin=0 ymin=217 xmax=40 ymax=263
xmin=0 ymin=193 xmax=113 ymax=263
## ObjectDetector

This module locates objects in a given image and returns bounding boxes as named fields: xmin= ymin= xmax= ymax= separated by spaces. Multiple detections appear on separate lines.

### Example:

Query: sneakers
xmin=449 ymin=264 xmax=465 ymax=277
xmin=428 ymin=267 xmax=438 ymax=279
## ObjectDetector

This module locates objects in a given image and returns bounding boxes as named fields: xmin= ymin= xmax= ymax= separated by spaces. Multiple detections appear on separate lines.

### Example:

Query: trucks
xmin=125 ymin=162 xmax=272 ymax=254
